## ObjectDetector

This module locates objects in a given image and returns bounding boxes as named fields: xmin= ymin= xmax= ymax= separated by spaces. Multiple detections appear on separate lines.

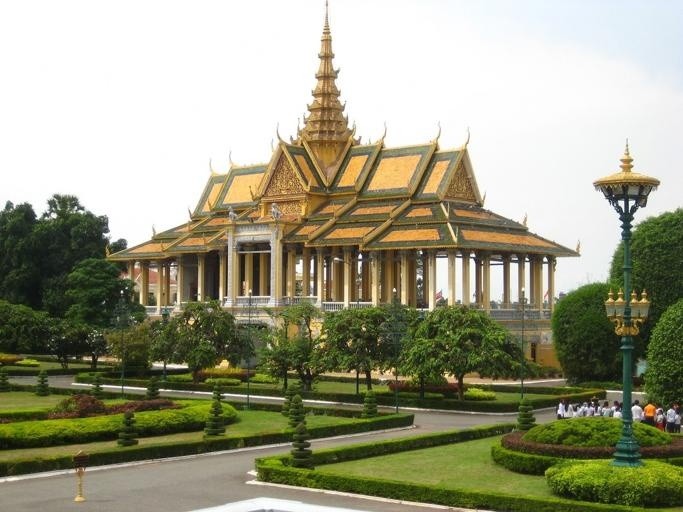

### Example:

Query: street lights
xmin=593 ymin=139 xmax=661 ymax=470
xmin=161 ymin=306 xmax=171 ymax=379
xmin=519 ymin=287 xmax=528 ymax=399
xmin=246 ymin=289 xmax=255 ymax=408
xmin=392 ymin=288 xmax=400 ymax=410
xmin=334 ymin=257 xmax=364 ymax=332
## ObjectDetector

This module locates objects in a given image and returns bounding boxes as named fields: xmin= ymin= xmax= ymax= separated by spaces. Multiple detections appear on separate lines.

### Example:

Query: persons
xmin=556 ymin=395 xmax=683 ymax=434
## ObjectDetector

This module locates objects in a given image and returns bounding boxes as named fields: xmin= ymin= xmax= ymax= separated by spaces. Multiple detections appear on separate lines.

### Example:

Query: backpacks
xmin=663 ymin=418 xmax=666 ymax=427
xmin=556 ymin=404 xmax=558 ymax=413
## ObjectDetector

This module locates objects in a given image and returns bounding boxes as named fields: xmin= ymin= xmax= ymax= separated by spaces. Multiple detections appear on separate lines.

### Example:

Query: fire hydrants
xmin=71 ymin=450 xmax=88 ymax=503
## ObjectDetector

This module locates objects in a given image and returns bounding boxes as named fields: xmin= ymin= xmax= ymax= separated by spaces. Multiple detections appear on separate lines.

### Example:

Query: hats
xmin=592 ymin=396 xmax=599 ymax=400
xmin=634 ymin=400 xmax=639 ymax=404
